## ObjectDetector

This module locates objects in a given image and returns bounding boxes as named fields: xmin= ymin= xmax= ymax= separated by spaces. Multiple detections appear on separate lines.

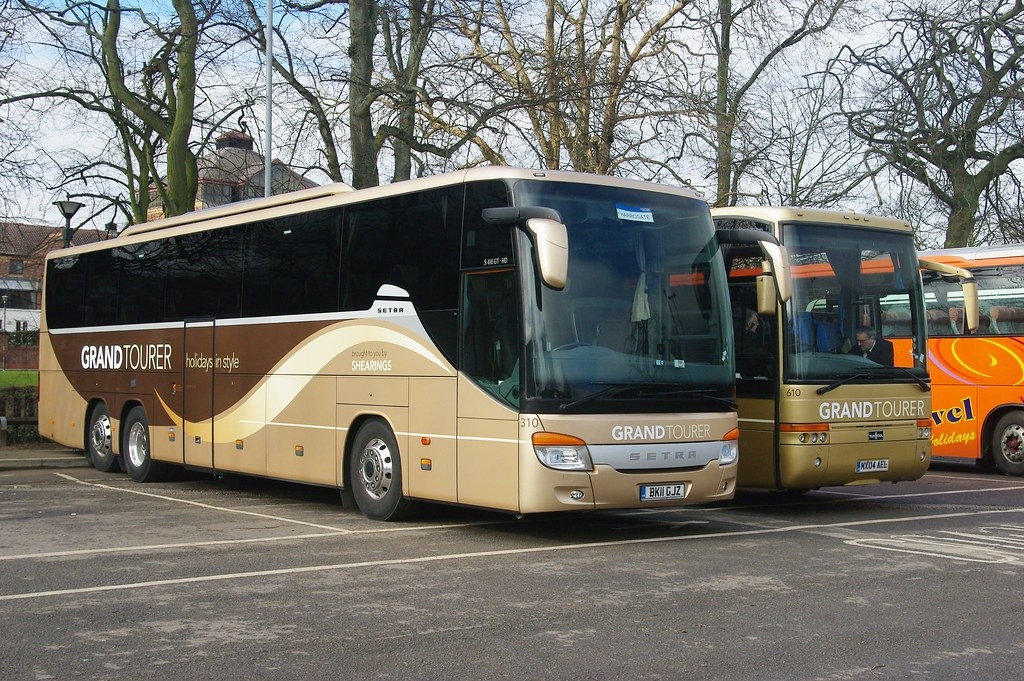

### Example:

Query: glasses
xmin=855 ymin=336 xmax=872 ymax=344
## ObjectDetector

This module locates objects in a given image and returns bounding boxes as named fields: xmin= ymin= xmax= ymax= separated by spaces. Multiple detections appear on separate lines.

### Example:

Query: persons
xmin=847 ymin=327 xmax=894 ymax=367
xmin=734 ymin=308 xmax=770 ymax=378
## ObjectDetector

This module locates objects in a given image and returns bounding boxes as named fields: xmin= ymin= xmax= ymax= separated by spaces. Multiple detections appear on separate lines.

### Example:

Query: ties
xmin=866 ymin=350 xmax=870 ymax=356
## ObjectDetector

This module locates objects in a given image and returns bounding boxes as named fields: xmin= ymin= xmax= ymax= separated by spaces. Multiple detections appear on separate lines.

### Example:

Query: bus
xmin=667 ymin=243 xmax=1024 ymax=477
xmin=705 ymin=205 xmax=980 ymax=496
xmin=37 ymin=164 xmax=791 ymax=522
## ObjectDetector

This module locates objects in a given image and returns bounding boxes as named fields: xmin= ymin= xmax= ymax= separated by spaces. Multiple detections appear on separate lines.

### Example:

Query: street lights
xmin=2 ymin=296 xmax=8 ymax=331
xmin=52 ymin=201 xmax=86 ymax=249
